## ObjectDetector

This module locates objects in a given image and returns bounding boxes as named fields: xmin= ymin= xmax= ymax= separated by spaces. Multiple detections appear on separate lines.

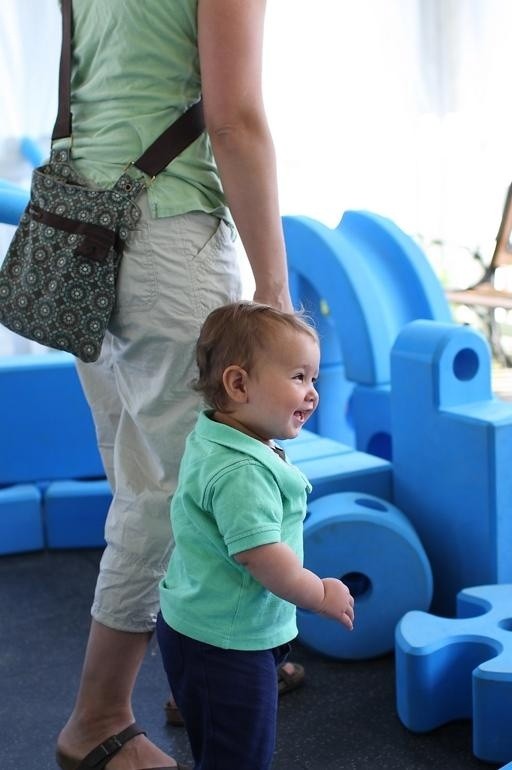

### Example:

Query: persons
xmin=155 ymin=302 xmax=355 ymax=769
xmin=51 ymin=0 xmax=305 ymax=769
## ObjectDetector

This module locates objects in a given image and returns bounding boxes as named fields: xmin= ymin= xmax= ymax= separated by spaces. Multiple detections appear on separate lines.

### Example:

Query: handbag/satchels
xmin=2 ymin=150 xmax=146 ymax=367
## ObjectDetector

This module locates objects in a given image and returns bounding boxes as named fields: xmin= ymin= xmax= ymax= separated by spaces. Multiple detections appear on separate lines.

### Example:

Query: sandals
xmin=165 ymin=657 xmax=309 ymax=728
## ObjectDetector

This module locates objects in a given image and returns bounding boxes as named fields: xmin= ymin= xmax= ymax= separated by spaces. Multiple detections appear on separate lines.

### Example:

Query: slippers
xmin=56 ymin=722 xmax=196 ymax=770
